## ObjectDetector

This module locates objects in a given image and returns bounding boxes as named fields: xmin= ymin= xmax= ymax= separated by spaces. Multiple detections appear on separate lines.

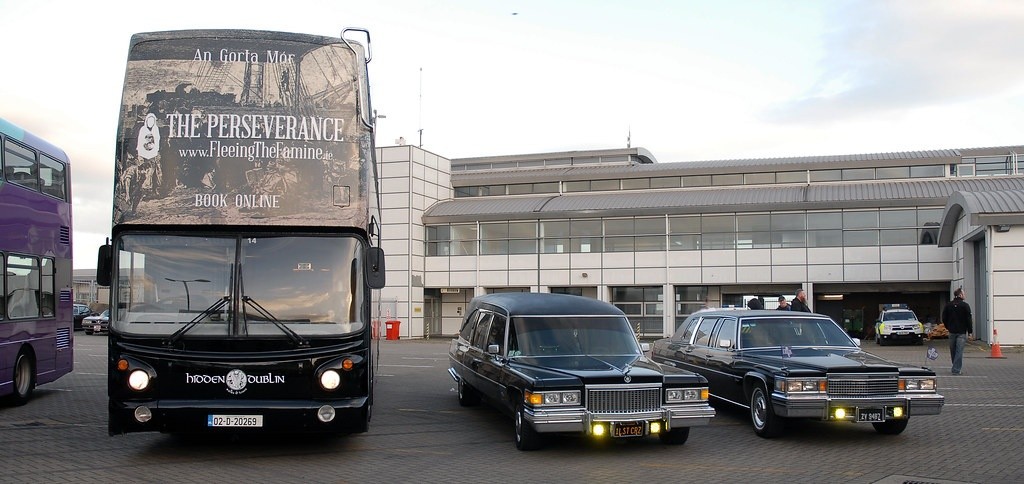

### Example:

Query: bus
xmin=0 ymin=118 xmax=76 ymax=409
xmin=96 ymin=26 xmax=388 ymax=450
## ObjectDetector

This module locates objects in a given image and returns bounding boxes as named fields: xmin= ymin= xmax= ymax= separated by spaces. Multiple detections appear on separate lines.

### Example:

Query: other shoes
xmin=952 ymin=371 xmax=963 ymax=375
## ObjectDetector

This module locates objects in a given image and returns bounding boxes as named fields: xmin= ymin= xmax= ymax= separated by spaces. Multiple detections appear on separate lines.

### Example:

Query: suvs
xmin=874 ymin=303 xmax=925 ymax=344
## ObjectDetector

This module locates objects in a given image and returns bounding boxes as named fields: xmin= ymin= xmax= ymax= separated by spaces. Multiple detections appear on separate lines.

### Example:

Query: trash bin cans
xmin=385 ymin=320 xmax=401 ymax=340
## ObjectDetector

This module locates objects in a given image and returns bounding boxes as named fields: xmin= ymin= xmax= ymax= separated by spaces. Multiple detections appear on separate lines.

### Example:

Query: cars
xmin=650 ymin=307 xmax=945 ymax=437
xmin=448 ymin=294 xmax=716 ymax=452
xmin=81 ymin=307 xmax=127 ymax=335
xmin=73 ymin=303 xmax=100 ymax=331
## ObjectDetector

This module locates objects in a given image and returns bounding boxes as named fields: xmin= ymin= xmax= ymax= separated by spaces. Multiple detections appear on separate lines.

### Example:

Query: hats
xmin=778 ymin=296 xmax=785 ymax=302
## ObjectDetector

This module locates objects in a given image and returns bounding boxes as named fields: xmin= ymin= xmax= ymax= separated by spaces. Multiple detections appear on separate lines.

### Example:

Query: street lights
xmin=163 ymin=276 xmax=212 ymax=311
xmin=416 ymin=62 xmax=430 ymax=150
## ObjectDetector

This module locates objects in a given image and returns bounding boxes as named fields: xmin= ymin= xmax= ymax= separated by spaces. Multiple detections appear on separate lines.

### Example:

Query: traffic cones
xmin=987 ymin=329 xmax=1008 ymax=359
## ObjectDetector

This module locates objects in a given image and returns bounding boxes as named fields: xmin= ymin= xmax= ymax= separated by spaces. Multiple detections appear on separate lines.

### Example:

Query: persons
xmin=115 ymin=163 xmax=151 ymax=215
xmin=776 ymin=296 xmax=791 ymax=311
xmin=941 ymin=288 xmax=973 ymax=374
xmin=791 ymin=289 xmax=812 ymax=312
xmin=748 ymin=298 xmax=763 ymax=310
